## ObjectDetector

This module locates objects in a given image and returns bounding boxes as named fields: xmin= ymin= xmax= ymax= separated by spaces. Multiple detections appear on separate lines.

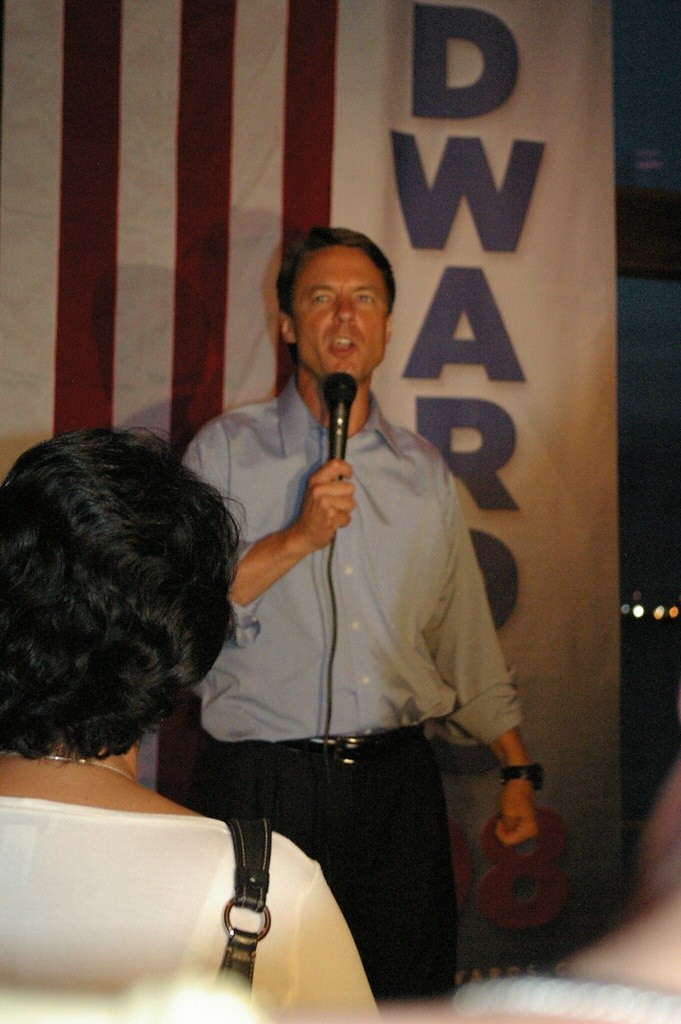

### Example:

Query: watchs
xmin=499 ymin=762 xmax=542 ymax=792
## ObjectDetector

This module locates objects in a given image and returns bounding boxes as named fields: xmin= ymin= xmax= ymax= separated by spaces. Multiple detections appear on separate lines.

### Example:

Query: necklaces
xmin=0 ymin=750 xmax=133 ymax=779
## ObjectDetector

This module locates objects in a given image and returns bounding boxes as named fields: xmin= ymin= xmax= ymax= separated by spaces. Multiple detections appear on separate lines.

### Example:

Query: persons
xmin=0 ymin=428 xmax=379 ymax=1024
xmin=176 ymin=228 xmax=542 ymax=1008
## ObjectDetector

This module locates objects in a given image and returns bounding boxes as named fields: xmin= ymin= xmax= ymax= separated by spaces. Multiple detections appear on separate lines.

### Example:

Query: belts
xmin=275 ymin=723 xmax=423 ymax=760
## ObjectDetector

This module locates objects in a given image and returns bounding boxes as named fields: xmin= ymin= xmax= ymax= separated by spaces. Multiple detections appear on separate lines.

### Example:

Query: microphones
xmin=322 ymin=369 xmax=359 ymax=482
